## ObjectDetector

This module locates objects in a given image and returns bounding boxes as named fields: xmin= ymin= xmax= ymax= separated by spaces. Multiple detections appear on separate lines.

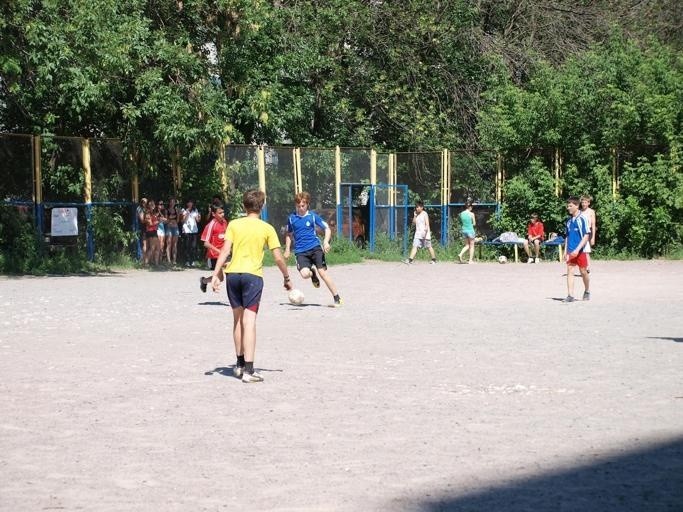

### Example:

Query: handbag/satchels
xmin=146 ymin=215 xmax=158 ymax=232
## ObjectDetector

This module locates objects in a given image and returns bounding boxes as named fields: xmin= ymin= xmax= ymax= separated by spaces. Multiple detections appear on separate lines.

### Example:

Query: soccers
xmin=288 ymin=289 xmax=305 ymax=305
xmin=498 ymin=255 xmax=507 ymax=264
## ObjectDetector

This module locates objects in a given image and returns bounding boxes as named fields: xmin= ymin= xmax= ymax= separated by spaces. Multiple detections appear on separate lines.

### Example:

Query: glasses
xmin=159 ymin=203 xmax=164 ymax=205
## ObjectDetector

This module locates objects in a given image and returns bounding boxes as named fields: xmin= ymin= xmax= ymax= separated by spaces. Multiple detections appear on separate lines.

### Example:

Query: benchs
xmin=475 ymin=241 xmax=563 ymax=263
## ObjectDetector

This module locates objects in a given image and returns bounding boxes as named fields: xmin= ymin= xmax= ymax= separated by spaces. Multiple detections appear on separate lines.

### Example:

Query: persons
xmin=210 ymin=187 xmax=293 ymax=382
xmin=401 ymin=201 xmax=439 ymax=266
xmin=282 ymin=190 xmax=343 ymax=310
xmin=524 ymin=211 xmax=546 ymax=263
xmin=561 ymin=195 xmax=592 ymax=304
xmin=456 ymin=202 xmax=479 ymax=266
xmin=580 ymin=194 xmax=596 ymax=274
xmin=135 ymin=194 xmax=229 ymax=293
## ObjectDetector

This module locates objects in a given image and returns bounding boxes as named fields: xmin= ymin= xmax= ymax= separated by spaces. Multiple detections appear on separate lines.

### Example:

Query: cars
xmin=277 ymin=208 xmax=365 ymax=248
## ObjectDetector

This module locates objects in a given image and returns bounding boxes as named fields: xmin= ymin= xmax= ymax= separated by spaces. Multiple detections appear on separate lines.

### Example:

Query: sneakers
xmin=310 ymin=268 xmax=320 ymax=288
xmin=401 ymin=257 xmax=439 ymax=265
xmin=527 ymin=256 xmax=541 ymax=264
xmin=200 ymin=277 xmax=208 ymax=293
xmin=185 ymin=260 xmax=196 ymax=267
xmin=563 ymin=291 xmax=590 ymax=302
xmin=457 ymin=254 xmax=475 ymax=264
xmin=233 ymin=366 xmax=264 ymax=383
xmin=335 ymin=297 xmax=343 ymax=307
